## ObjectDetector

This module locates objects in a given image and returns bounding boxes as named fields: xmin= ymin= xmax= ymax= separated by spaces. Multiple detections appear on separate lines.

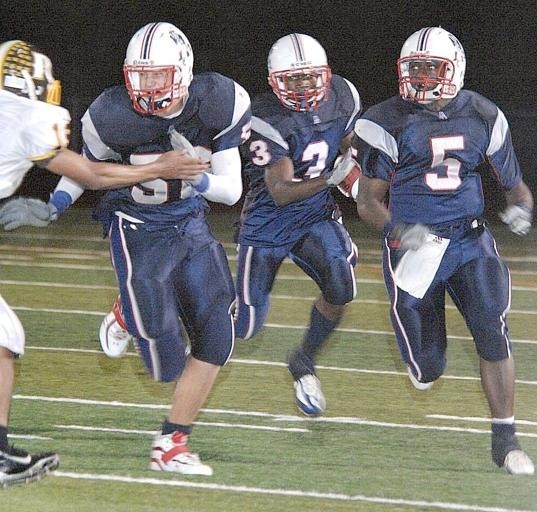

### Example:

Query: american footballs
xmin=333 ymin=154 xmax=362 ymax=197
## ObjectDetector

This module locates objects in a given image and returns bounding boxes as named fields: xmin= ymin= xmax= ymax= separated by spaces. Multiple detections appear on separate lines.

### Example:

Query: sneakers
xmin=504 ymin=449 xmax=534 ymax=474
xmin=99 ymin=294 xmax=133 ymax=358
xmin=407 ymin=364 xmax=434 ymax=390
xmin=8 ymin=444 xmax=60 ymax=486
xmin=149 ymin=429 xmax=213 ymax=476
xmin=287 ymin=349 xmax=327 ymax=417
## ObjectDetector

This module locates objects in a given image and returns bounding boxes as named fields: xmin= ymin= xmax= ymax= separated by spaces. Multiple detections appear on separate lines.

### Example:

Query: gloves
xmin=323 ymin=147 xmax=354 ymax=188
xmin=0 ymin=196 xmax=57 ymax=231
xmin=170 ymin=129 xmax=203 ymax=188
xmin=391 ymin=222 xmax=429 ymax=251
xmin=498 ymin=202 xmax=533 ymax=237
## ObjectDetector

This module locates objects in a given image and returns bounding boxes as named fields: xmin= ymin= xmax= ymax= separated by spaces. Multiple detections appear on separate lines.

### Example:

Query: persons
xmin=352 ymin=24 xmax=535 ymax=480
xmin=228 ymin=29 xmax=364 ymax=418
xmin=0 ymin=39 xmax=213 ymax=486
xmin=1 ymin=20 xmax=254 ymax=477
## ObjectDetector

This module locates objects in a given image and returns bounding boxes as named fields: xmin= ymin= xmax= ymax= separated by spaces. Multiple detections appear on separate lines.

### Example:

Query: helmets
xmin=397 ymin=27 xmax=467 ymax=104
xmin=0 ymin=39 xmax=62 ymax=105
xmin=267 ymin=33 xmax=333 ymax=112
xmin=124 ymin=21 xmax=195 ymax=115
